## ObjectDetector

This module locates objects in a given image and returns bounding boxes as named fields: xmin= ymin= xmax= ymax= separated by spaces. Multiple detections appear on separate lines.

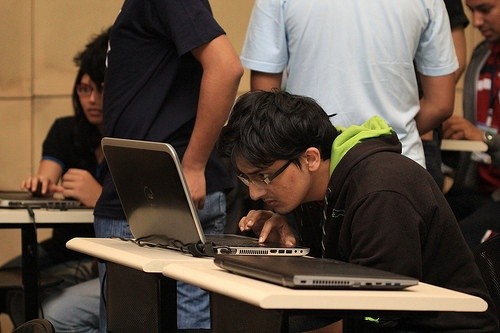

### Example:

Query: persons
xmin=415 ymin=0 xmax=500 ymax=250
xmin=240 ymin=0 xmax=459 ymax=170
xmin=0 ymin=27 xmax=110 ymax=333
xmin=217 ymin=90 xmax=500 ymax=333
xmin=93 ymin=0 xmax=245 ymax=333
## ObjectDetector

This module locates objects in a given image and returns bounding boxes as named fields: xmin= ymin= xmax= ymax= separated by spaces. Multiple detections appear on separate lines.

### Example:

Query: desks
xmin=66 ymin=234 xmax=487 ymax=333
xmin=0 ymin=208 xmax=95 ymax=324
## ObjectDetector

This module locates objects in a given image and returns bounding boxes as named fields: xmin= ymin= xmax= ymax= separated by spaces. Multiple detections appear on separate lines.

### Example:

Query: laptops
xmin=214 ymin=254 xmax=419 ymax=290
xmin=101 ymin=137 xmax=310 ymax=257
xmin=0 ymin=190 xmax=80 ymax=210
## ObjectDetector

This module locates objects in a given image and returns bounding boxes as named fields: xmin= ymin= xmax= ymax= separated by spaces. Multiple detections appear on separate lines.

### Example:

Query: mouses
xmin=28 ymin=180 xmax=48 ymax=198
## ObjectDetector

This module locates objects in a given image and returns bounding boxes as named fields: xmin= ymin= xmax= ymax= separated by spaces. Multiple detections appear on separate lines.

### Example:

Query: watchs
xmin=483 ymin=129 xmax=495 ymax=148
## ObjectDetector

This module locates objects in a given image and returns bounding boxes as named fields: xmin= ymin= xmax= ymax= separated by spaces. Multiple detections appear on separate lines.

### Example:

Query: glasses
xmin=78 ymin=84 xmax=103 ymax=94
xmin=237 ymin=149 xmax=305 ymax=185
